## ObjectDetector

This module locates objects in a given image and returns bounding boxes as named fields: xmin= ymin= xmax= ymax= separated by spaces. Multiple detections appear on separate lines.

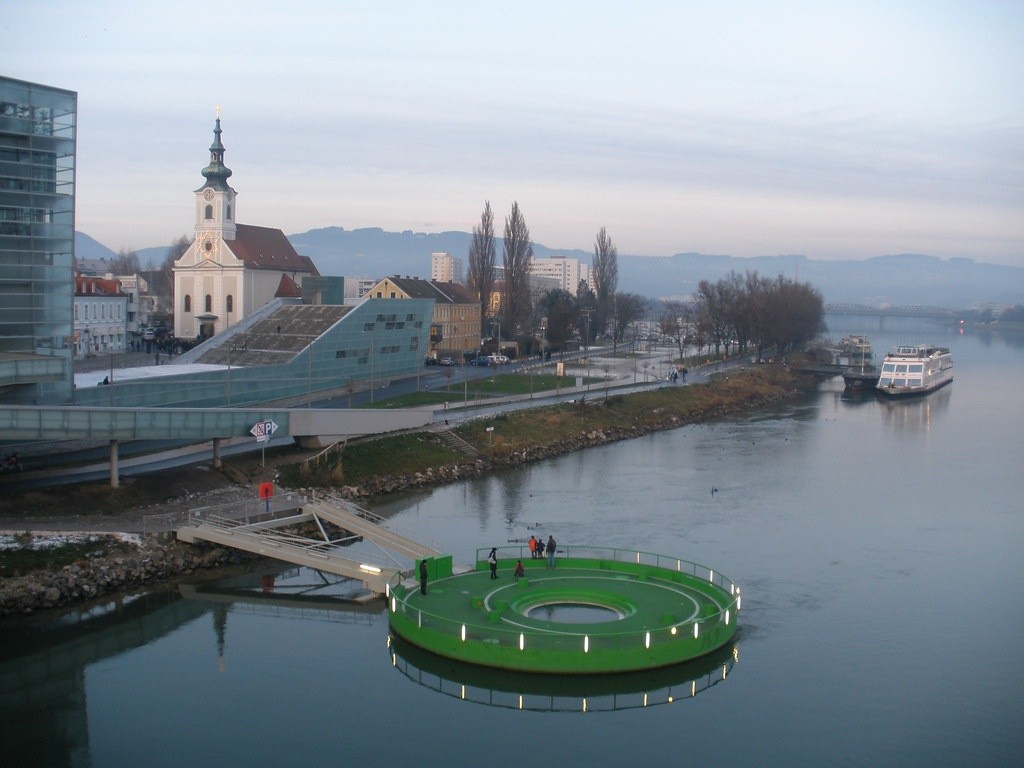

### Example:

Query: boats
xmin=837 ymin=334 xmax=956 ymax=396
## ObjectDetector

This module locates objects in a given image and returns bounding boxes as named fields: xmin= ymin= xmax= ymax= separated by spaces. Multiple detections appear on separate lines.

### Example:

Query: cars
xmin=467 ymin=355 xmax=510 ymax=366
xmin=441 ymin=356 xmax=456 ymax=366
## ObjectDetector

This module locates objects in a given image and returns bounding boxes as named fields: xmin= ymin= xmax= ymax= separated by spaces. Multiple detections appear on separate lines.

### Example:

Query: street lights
xmin=491 ymin=322 xmax=501 ymax=354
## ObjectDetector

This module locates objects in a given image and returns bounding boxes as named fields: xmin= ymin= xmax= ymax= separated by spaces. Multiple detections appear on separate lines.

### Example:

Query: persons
xmin=103 ymin=376 xmax=109 ymax=384
xmin=426 ymin=355 xmax=437 ymax=369
xmin=668 ymin=372 xmax=678 ymax=382
xmin=537 ymin=539 xmax=545 ymax=558
xmin=420 ymin=559 xmax=428 ymax=595
xmin=488 ymin=548 xmax=499 ymax=579
xmin=546 ymin=535 xmax=556 ymax=569
xmin=528 ymin=535 xmax=537 ymax=559
xmin=514 ymin=560 xmax=524 ymax=583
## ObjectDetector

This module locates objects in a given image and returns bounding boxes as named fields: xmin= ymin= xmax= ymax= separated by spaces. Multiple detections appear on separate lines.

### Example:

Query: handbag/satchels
xmin=488 ymin=553 xmax=497 ymax=565
